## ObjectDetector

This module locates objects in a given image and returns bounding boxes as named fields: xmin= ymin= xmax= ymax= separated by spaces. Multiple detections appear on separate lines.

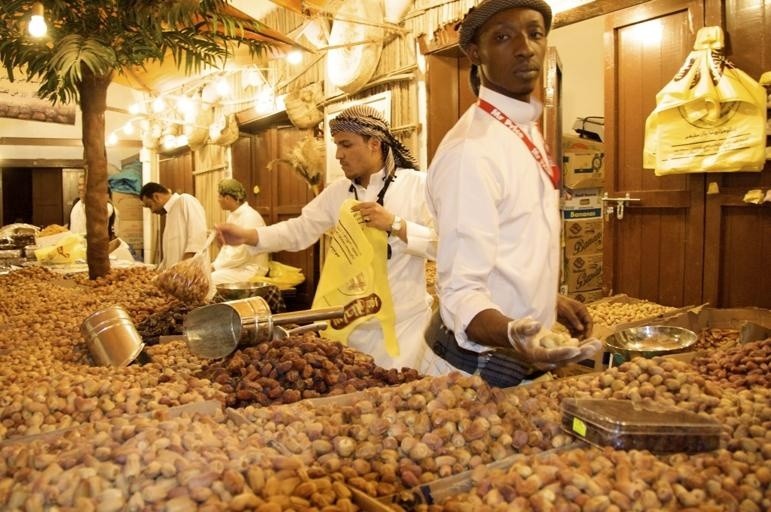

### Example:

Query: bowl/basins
xmin=602 ymin=325 xmax=698 ymax=362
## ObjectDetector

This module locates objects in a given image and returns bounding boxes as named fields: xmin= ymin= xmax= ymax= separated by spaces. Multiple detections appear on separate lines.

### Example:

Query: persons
xmin=138 ymin=181 xmax=210 ymax=268
xmin=66 ymin=174 xmax=116 ymax=243
xmin=422 ymin=0 xmax=596 ymax=387
xmin=208 ymin=105 xmax=438 ymax=374
xmin=206 ymin=179 xmax=267 ymax=301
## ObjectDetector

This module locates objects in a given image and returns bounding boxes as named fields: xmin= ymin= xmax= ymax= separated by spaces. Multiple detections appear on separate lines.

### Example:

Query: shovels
xmin=183 ymin=294 xmax=344 ymax=361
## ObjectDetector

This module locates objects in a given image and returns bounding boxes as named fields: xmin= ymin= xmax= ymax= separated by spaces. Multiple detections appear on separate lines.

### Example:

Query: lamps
xmin=28 ymin=1 xmax=47 ymax=39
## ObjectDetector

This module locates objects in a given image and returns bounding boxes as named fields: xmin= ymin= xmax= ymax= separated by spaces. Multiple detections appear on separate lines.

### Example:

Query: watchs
xmin=390 ymin=215 xmax=401 ymax=237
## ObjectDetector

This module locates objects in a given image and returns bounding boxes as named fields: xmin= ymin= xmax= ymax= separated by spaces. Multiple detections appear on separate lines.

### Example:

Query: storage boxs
xmin=562 ymin=132 xmax=604 ymax=302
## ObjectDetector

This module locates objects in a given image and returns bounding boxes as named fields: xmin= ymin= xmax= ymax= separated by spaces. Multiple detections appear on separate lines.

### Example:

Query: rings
xmin=364 ymin=215 xmax=370 ymax=222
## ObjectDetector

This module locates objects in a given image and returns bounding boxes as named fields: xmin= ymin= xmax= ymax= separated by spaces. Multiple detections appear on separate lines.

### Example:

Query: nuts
xmin=0 ymin=265 xmax=771 ymax=512
